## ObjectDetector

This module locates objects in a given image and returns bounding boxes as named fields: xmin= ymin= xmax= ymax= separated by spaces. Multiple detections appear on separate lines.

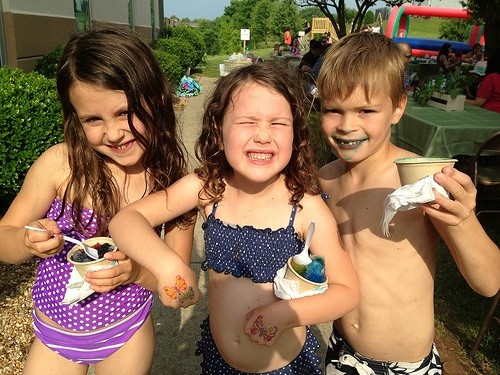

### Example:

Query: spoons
xmin=293 ymin=221 xmax=316 ymax=265
xmin=25 ymin=225 xmax=99 ymax=260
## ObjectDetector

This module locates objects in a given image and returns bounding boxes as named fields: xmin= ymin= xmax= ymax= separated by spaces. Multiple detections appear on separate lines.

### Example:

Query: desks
xmin=406 ymin=64 xmax=474 ymax=75
xmin=389 ymin=102 xmax=500 ymax=157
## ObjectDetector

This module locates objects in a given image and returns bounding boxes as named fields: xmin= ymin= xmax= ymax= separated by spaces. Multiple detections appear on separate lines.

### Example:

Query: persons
xmin=317 ymin=32 xmax=500 ymax=375
xmin=252 ymin=54 xmax=263 ymax=64
xmin=467 ymin=48 xmax=500 ymax=167
xmin=361 ymin=21 xmax=383 ymax=34
xmin=298 ymin=36 xmax=332 ymax=95
xmin=465 ymin=43 xmax=484 ymax=62
xmin=0 ymin=26 xmax=198 ymax=375
xmin=437 ymin=43 xmax=474 ymax=99
xmin=109 ymin=61 xmax=360 ymax=375
xmin=286 ymin=39 xmax=301 ymax=57
xmin=284 ymin=26 xmax=291 ymax=51
xmin=272 ymin=44 xmax=281 ymax=57
xmin=396 ymin=42 xmax=429 ymax=97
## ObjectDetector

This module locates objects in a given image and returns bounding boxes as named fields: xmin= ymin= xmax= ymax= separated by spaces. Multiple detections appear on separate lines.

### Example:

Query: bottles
xmin=472 ymin=55 xmax=476 ymax=65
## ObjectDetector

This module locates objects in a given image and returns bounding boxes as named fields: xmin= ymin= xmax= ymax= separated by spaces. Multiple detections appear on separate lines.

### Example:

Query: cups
xmin=393 ymin=157 xmax=459 ymax=205
xmin=425 ymin=53 xmax=429 ymax=59
xmin=284 ymin=256 xmax=329 ymax=295
xmin=66 ymin=236 xmax=119 ymax=278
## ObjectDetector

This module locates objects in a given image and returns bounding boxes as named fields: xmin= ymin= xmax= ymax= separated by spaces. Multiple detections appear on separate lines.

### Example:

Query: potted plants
xmin=416 ymin=69 xmax=466 ymax=111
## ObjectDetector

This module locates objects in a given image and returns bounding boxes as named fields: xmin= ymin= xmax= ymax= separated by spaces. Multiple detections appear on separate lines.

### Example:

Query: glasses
xmin=404 ymin=54 xmax=412 ymax=58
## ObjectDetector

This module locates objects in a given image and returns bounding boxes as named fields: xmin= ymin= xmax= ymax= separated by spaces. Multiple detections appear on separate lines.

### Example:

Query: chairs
xmin=299 ymin=71 xmax=319 ymax=118
xmin=453 ymin=131 xmax=500 ymax=215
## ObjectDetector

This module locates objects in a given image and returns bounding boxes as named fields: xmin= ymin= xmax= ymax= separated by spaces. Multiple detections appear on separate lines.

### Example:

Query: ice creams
xmin=71 ymin=242 xmax=115 ymax=262
xmin=290 ymin=255 xmax=328 ymax=284
